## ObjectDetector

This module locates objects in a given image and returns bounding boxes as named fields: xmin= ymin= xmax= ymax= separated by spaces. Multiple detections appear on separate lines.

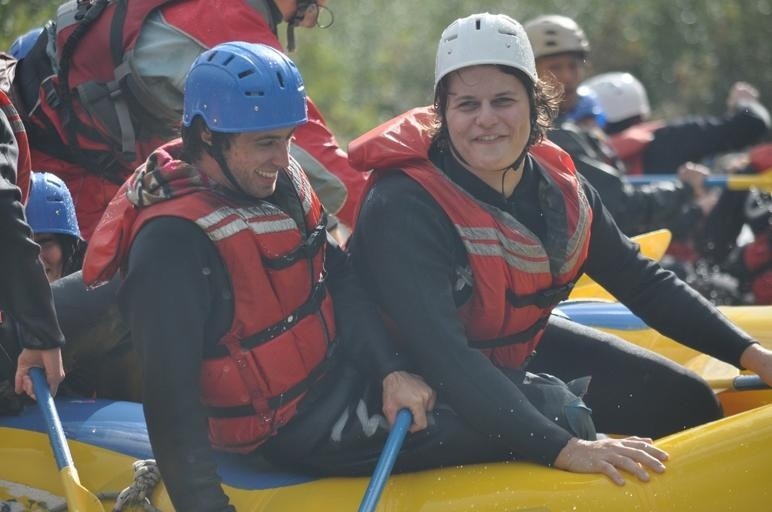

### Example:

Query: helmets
xmin=24 ymin=170 xmax=81 ymax=240
xmin=182 ymin=41 xmax=308 ymax=133
xmin=578 ymin=71 xmax=650 ymax=124
xmin=522 ymin=14 xmax=587 ymax=62
xmin=433 ymin=12 xmax=538 ymax=91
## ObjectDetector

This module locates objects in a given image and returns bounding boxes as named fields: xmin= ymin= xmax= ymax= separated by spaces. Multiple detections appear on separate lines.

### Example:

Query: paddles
xmin=26 ymin=366 xmax=105 ymax=512
xmin=623 ymin=171 xmax=771 ymax=195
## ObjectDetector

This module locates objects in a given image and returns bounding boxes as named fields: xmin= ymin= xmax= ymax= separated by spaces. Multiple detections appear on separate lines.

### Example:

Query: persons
xmin=125 ymin=40 xmax=596 ymax=512
xmin=0 ymin=1 xmax=373 ymax=228
xmin=24 ymin=171 xmax=89 ymax=284
xmin=352 ymin=14 xmax=772 ymax=512
xmin=0 ymin=47 xmax=120 ymax=420
xmin=522 ymin=15 xmax=772 ymax=304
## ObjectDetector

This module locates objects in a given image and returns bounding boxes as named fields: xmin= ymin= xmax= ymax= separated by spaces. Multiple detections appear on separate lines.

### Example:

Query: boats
xmin=3 ymin=299 xmax=772 ymax=508
